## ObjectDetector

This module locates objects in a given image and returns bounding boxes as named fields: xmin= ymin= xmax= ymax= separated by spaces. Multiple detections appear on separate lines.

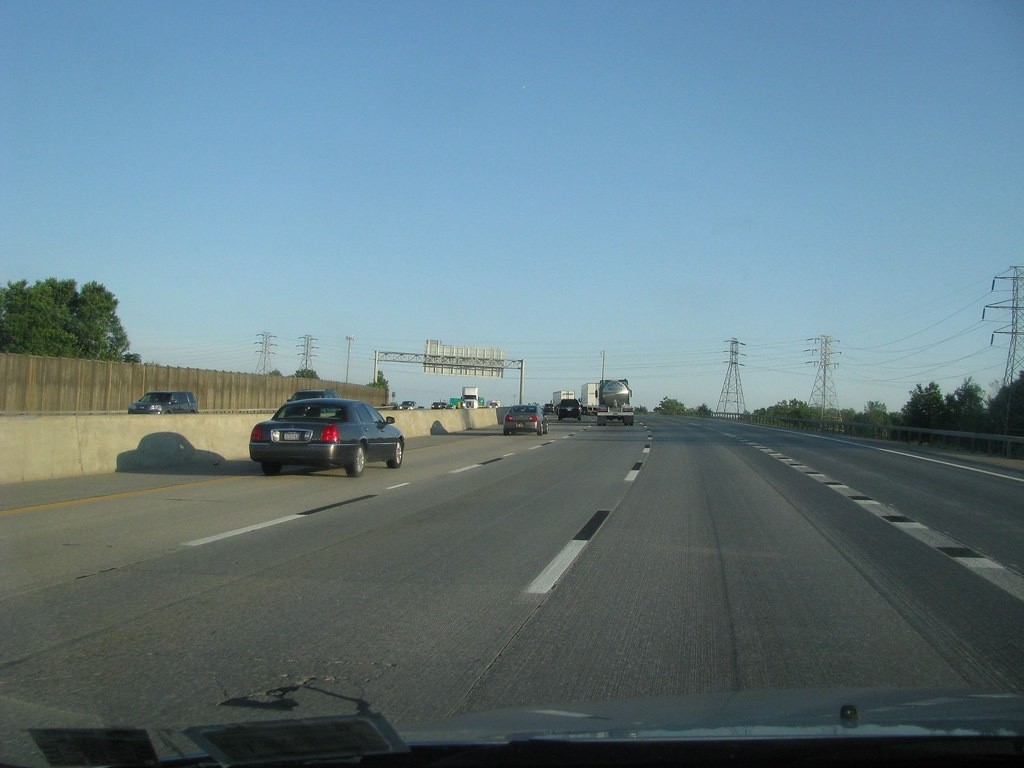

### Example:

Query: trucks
xmin=461 ymin=387 xmax=480 ymax=409
xmin=595 ymin=378 xmax=634 ymax=426
xmin=550 ymin=389 xmax=575 ymax=405
xmin=578 ymin=382 xmax=600 ymax=416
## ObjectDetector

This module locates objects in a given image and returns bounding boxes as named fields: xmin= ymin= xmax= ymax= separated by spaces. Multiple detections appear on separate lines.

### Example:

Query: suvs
xmin=128 ymin=391 xmax=199 ymax=414
xmin=556 ymin=398 xmax=582 ymax=421
xmin=286 ymin=388 xmax=341 ymax=413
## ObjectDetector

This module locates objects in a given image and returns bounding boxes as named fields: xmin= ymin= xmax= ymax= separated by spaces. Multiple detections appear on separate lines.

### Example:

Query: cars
xmin=502 ymin=405 xmax=548 ymax=436
xmin=248 ymin=397 xmax=408 ymax=477
xmin=489 ymin=402 xmax=499 ymax=408
xmin=398 ymin=400 xmax=418 ymax=410
xmin=431 ymin=402 xmax=454 ymax=410
xmin=542 ymin=403 xmax=555 ymax=414
